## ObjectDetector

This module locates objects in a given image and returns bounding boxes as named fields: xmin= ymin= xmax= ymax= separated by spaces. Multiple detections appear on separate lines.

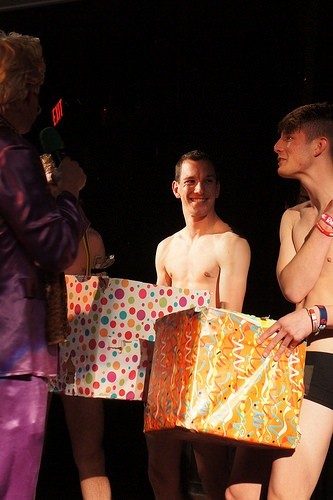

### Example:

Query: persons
xmin=144 ymin=148 xmax=250 ymax=500
xmin=0 ymin=31 xmax=112 ymax=500
xmin=224 ymin=102 xmax=333 ymax=500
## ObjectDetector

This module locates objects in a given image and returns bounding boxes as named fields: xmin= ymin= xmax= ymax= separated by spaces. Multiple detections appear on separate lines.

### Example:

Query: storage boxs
xmin=48 ymin=274 xmax=307 ymax=450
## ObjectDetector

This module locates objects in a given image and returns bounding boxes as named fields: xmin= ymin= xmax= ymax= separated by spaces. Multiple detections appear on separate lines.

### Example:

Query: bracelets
xmin=320 ymin=212 xmax=333 ymax=229
xmin=314 ymin=304 xmax=327 ymax=336
xmin=301 ymin=305 xmax=318 ymax=333
xmin=315 ymin=222 xmax=333 ymax=237
